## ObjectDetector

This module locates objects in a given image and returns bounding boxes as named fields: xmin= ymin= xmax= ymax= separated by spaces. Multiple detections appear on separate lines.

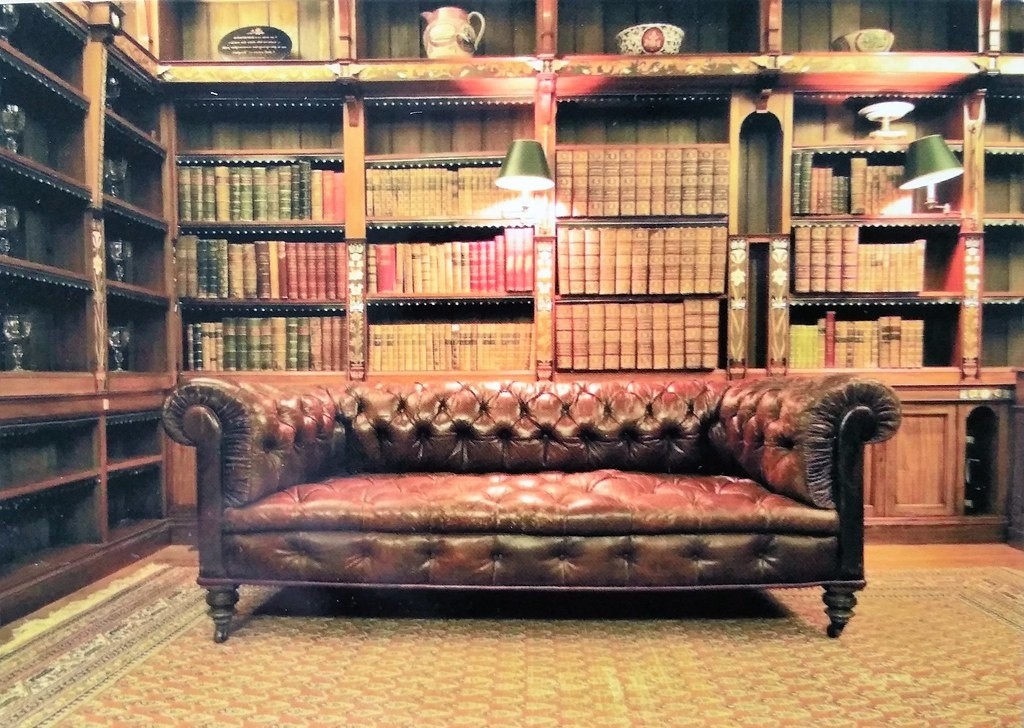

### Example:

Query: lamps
xmin=495 ymin=140 xmax=556 ymax=224
xmin=899 ymin=134 xmax=965 ymax=215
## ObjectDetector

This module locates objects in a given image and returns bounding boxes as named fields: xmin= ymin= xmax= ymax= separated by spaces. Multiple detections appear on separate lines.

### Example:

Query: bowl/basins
xmin=615 ymin=23 xmax=685 ymax=56
xmin=831 ymin=29 xmax=895 ymax=52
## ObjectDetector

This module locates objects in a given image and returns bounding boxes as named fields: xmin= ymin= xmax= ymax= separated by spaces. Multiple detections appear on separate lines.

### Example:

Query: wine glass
xmin=106 ymin=158 xmax=128 ymax=197
xmin=0 ymin=206 xmax=20 ymax=256
xmin=0 ymin=4 xmax=20 ymax=43
xmin=108 ymin=241 xmax=132 ymax=281
xmin=1 ymin=103 xmax=25 ymax=153
xmin=3 ymin=314 xmax=31 ymax=372
xmin=108 ymin=327 xmax=130 ymax=372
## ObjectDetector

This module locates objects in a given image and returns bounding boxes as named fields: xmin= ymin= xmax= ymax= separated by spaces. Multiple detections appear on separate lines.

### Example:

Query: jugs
xmin=421 ymin=6 xmax=485 ymax=58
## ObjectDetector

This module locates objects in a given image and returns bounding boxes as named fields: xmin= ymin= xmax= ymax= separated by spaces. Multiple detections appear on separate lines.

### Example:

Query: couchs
xmin=161 ymin=375 xmax=903 ymax=642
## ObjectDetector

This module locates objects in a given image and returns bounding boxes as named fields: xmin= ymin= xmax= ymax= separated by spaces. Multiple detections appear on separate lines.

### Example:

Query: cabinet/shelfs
xmin=0 ymin=0 xmax=1024 ymax=627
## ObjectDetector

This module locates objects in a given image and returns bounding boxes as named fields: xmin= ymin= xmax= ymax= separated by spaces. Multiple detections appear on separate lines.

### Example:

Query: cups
xmin=106 ymin=77 xmax=121 ymax=108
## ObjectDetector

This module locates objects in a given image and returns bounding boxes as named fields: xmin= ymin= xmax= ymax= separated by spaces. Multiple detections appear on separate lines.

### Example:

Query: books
xmin=176 ymin=165 xmax=348 ymax=372
xmin=365 ymin=168 xmax=533 ymax=372
xmin=556 ymin=149 xmax=730 ymax=369
xmin=791 ymin=149 xmax=928 ymax=371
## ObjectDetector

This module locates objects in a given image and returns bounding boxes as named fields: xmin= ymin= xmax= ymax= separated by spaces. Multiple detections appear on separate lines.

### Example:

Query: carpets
xmin=0 ymin=563 xmax=1024 ymax=728
